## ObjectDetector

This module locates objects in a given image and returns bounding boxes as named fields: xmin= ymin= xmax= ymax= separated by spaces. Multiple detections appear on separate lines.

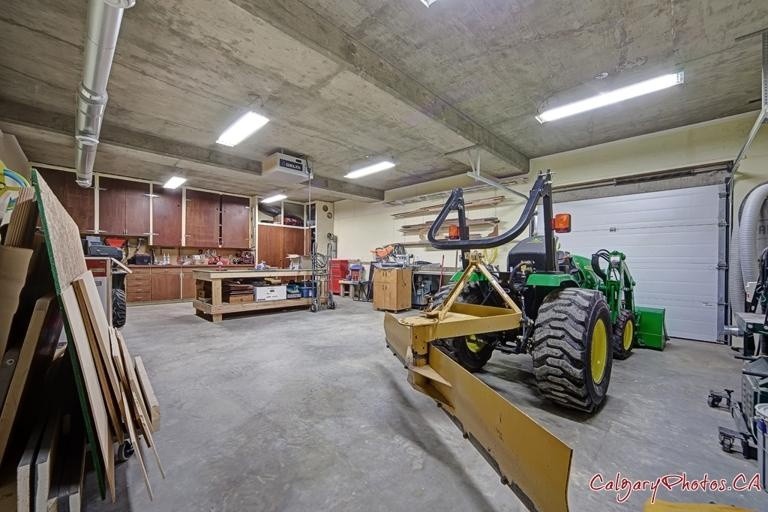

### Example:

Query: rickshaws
xmin=310 ymin=242 xmax=335 ymax=312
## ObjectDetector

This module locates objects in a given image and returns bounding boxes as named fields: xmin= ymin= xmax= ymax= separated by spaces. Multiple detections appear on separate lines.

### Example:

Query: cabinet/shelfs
xmin=152 ymin=267 xmax=181 ymax=302
xmin=251 ymin=195 xmax=304 ymax=231
xmin=182 ymin=266 xmax=217 ymax=302
xmin=153 ymin=183 xmax=182 ymax=250
xmin=29 ymin=163 xmax=96 ymax=236
xmin=127 ymin=264 xmax=151 ymax=302
xmin=187 ymin=189 xmax=249 ymax=249
xmin=303 ymin=201 xmax=333 ymax=295
xmin=373 ymin=267 xmax=412 ymax=313
xmin=327 ymin=259 xmax=348 ymax=294
xmin=98 ymin=174 xmax=151 ymax=237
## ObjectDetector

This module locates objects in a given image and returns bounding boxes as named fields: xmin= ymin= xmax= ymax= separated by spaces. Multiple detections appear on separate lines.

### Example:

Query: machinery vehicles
xmin=384 ymin=168 xmax=669 ymax=511
xmin=78 ymin=234 xmax=128 ymax=327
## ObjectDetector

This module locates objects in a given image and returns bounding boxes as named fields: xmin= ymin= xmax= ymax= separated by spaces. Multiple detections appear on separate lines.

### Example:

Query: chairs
xmin=338 ymin=265 xmax=364 ymax=301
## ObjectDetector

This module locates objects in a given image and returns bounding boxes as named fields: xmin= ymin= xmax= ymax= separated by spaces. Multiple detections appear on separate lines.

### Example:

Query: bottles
xmin=163 ymin=252 xmax=171 ymax=264
xmin=402 ymin=257 xmax=406 ymax=269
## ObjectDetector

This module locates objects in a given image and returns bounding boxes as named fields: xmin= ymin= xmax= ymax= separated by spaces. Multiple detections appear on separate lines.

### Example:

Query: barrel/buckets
xmin=298 ymin=287 xmax=316 ymax=297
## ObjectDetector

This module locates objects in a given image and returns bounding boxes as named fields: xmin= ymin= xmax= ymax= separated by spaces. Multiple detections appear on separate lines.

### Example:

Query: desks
xmin=192 ymin=269 xmax=330 ymax=323
